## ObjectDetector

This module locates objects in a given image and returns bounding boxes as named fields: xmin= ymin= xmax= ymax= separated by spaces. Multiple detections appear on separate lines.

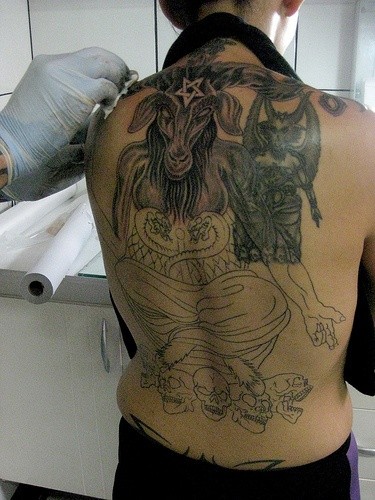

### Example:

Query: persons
xmin=0 ymin=47 xmax=131 ymax=203
xmin=84 ymin=0 xmax=375 ymax=500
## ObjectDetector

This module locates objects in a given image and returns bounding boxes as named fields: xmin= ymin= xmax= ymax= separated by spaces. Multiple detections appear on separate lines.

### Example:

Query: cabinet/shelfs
xmin=0 ymin=269 xmax=133 ymax=500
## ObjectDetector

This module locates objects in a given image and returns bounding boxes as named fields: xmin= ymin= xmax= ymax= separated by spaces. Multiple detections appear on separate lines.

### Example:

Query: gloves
xmin=1 ymin=111 xmax=96 ymax=201
xmin=0 ymin=47 xmax=131 ymax=185
